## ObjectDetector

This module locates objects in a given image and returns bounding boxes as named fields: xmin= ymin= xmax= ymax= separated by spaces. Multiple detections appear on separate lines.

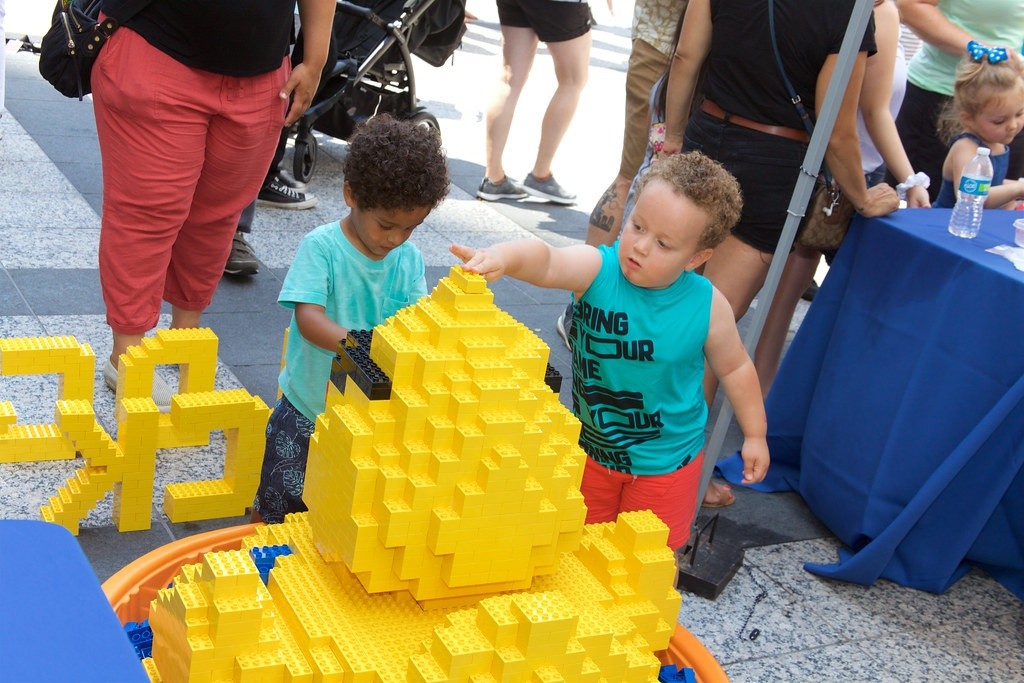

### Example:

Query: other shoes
xmin=476 ymin=176 xmax=528 ymax=200
xmin=556 ymin=310 xmax=573 ymax=353
xmin=521 ymin=172 xmax=577 ymax=204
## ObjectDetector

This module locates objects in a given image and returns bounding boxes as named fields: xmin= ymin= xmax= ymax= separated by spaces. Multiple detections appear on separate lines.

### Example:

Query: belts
xmin=703 ymin=103 xmax=812 ymax=141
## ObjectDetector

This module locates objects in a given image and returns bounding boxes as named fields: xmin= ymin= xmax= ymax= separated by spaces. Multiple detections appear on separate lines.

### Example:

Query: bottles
xmin=949 ymin=146 xmax=993 ymax=239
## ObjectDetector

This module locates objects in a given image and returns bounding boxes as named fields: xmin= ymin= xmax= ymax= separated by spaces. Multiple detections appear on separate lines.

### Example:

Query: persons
xmin=90 ymin=0 xmax=1024 ymax=509
xmin=449 ymin=151 xmax=771 ymax=589
xmin=247 ymin=113 xmax=451 ymax=528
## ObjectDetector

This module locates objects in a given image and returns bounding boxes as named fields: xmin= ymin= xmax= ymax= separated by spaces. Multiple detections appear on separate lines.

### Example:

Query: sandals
xmin=702 ymin=480 xmax=736 ymax=507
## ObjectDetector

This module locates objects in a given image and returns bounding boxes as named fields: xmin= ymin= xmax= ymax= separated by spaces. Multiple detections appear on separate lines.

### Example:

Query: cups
xmin=1013 ymin=219 xmax=1024 ymax=247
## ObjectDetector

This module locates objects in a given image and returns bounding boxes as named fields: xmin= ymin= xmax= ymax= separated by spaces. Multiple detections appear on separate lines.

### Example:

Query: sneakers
xmin=257 ymin=178 xmax=318 ymax=210
xmin=222 ymin=230 xmax=259 ymax=276
xmin=103 ymin=356 xmax=176 ymax=410
xmin=275 ymin=169 xmax=309 ymax=192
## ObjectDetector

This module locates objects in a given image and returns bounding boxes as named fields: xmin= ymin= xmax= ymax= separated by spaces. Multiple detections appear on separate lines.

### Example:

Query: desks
xmin=0 ymin=519 xmax=152 ymax=683
xmin=714 ymin=208 xmax=1024 ymax=607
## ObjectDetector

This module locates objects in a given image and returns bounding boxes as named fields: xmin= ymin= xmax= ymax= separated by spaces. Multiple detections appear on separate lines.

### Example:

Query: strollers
xmin=291 ymin=0 xmax=468 ymax=184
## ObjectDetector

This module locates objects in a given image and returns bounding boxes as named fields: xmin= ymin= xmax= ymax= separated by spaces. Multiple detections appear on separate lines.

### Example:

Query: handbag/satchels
xmin=799 ymin=184 xmax=852 ymax=250
xmin=38 ymin=1 xmax=118 ymax=101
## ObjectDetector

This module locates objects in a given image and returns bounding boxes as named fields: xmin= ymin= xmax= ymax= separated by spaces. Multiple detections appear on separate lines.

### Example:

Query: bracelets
xmin=896 ymin=171 xmax=931 ymax=200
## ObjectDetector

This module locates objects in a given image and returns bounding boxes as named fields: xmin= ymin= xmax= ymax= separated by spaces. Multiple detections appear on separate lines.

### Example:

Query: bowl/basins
xmin=101 ymin=521 xmax=730 ymax=682
